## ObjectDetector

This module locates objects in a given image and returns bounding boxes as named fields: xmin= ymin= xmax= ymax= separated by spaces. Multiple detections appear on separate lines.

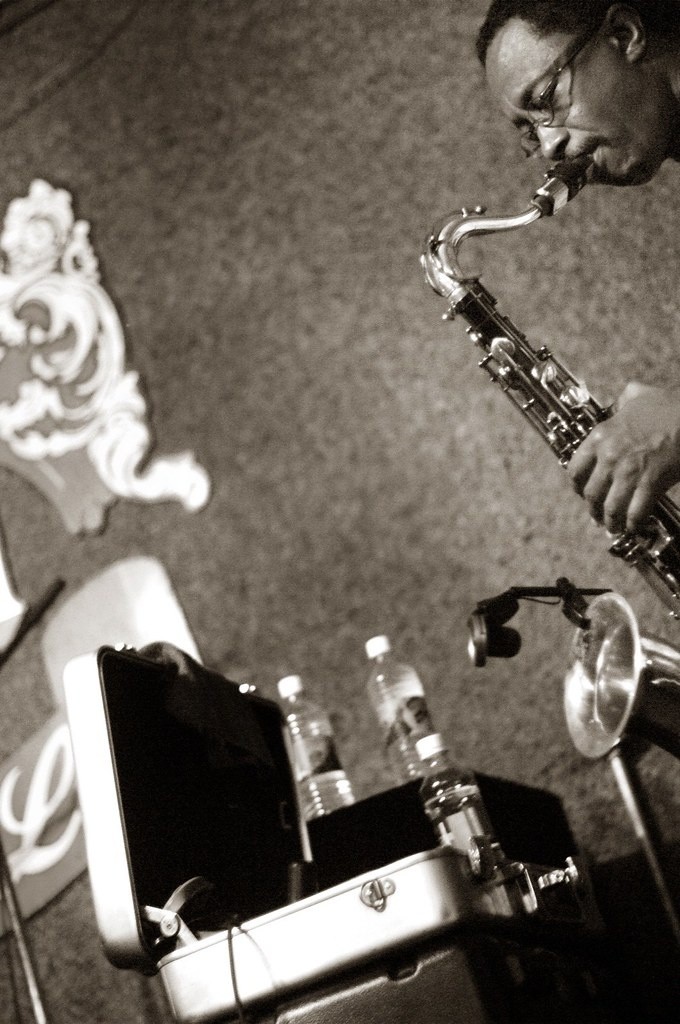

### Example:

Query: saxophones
xmin=418 ymin=151 xmax=679 ymax=761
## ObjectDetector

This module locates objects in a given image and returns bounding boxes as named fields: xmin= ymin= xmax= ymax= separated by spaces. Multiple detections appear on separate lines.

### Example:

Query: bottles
xmin=364 ymin=635 xmax=440 ymax=784
xmin=276 ymin=674 xmax=356 ymax=821
xmin=414 ymin=731 xmax=493 ymax=854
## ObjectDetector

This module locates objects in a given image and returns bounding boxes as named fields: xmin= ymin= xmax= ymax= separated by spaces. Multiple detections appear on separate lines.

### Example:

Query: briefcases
xmin=61 ymin=642 xmax=586 ymax=1022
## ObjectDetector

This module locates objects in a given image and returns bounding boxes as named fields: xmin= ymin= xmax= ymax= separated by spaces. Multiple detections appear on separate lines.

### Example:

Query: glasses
xmin=518 ymin=23 xmax=602 ymax=160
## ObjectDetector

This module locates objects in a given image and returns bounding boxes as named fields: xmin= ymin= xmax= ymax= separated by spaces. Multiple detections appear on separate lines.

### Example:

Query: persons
xmin=464 ymin=0 xmax=680 ymax=534
